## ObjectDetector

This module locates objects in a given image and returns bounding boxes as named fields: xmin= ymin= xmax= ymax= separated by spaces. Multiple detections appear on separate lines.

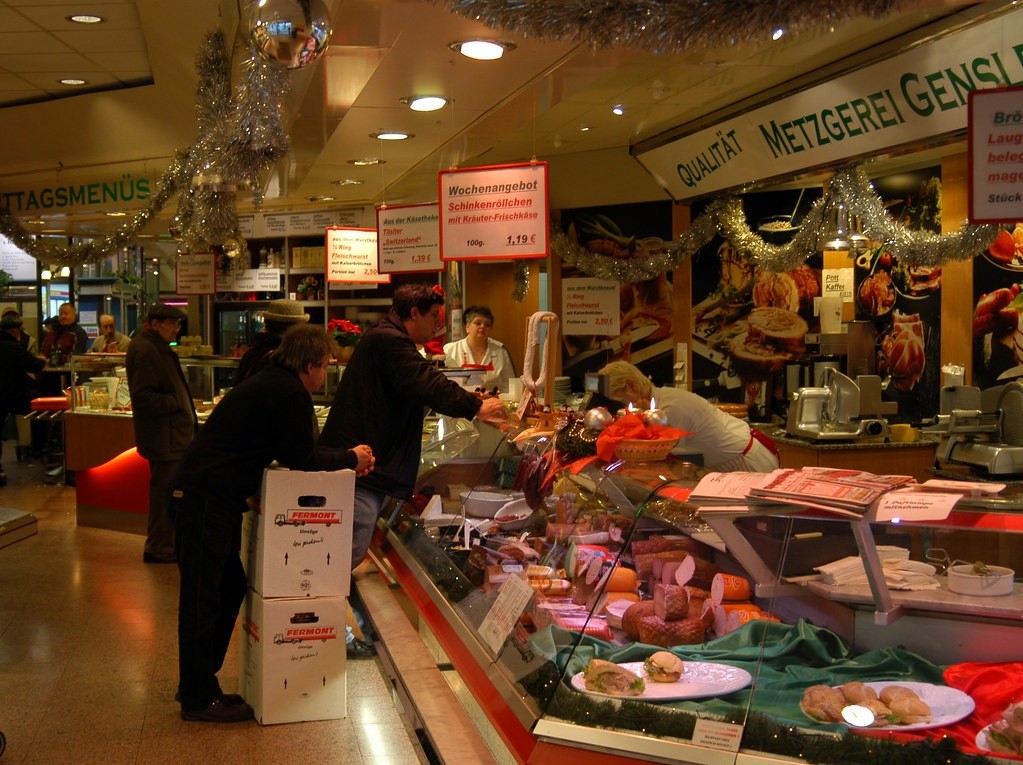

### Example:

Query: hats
xmin=149 ymin=304 xmax=186 ymax=319
xmin=262 ymin=298 xmax=311 ymax=322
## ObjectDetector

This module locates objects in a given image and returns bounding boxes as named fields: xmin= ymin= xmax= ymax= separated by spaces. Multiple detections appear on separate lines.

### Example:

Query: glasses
xmin=160 ymin=323 xmax=184 ymax=332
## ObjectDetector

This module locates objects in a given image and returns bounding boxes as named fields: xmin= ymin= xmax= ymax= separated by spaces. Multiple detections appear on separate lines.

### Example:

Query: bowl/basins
xmin=947 ymin=565 xmax=1015 ymax=596
xmin=756 ymin=216 xmax=802 ymax=245
xmin=569 ymin=531 xmax=610 ymax=544
xmin=890 ymin=267 xmax=932 ymax=302
xmin=494 ymin=498 xmax=534 ymax=530
xmin=857 ymin=273 xmax=897 ymax=316
xmin=980 ymin=251 xmax=1023 ymax=276
xmin=459 ymin=492 xmax=514 ymax=518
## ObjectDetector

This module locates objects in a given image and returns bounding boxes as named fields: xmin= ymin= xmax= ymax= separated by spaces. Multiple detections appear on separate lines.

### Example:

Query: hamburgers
xmin=800 ymin=680 xmax=933 ymax=728
xmin=984 ymin=702 xmax=1023 ymax=759
xmin=643 ymin=650 xmax=683 ymax=682
xmin=580 ymin=658 xmax=645 ymax=697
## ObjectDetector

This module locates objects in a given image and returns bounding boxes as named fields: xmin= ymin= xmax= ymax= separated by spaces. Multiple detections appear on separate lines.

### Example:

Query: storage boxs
xmin=293 ymin=247 xmax=325 ymax=269
xmin=240 ymin=466 xmax=356 ymax=723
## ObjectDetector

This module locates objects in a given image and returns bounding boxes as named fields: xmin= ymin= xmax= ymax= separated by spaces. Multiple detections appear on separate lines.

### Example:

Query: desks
xmin=774 ymin=429 xmax=935 ymax=478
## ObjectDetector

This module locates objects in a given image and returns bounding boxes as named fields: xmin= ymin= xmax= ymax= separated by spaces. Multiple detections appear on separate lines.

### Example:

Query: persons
xmin=0 ymin=303 xmax=132 ymax=455
xmin=234 ymin=300 xmax=311 ymax=388
xmin=596 ymin=362 xmax=782 ymax=476
xmin=315 ymin=282 xmax=509 ymax=661
xmin=443 ymin=305 xmax=517 ymax=393
xmin=174 ymin=324 xmax=375 ymax=722
xmin=126 ymin=305 xmax=197 ymax=564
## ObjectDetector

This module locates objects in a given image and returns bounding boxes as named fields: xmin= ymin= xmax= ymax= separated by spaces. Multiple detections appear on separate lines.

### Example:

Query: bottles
xmin=268 ymin=248 xmax=274 ymax=268
xmin=556 ymin=497 xmax=572 ymax=524
xmin=259 ymin=247 xmax=267 ymax=268
xmin=235 ymin=314 xmax=248 ymax=345
xmin=50 ymin=345 xmax=56 ymax=368
xmin=274 ymin=252 xmax=280 ymax=268
xmin=56 ymin=346 xmax=62 ymax=366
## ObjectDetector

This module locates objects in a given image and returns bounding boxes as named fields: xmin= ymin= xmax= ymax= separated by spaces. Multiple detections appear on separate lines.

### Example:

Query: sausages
xmin=484 ymin=511 xmax=781 ymax=639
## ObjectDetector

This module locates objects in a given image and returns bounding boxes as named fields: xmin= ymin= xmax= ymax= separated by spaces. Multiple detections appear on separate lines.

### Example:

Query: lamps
xmin=822 ymin=195 xmax=870 ymax=253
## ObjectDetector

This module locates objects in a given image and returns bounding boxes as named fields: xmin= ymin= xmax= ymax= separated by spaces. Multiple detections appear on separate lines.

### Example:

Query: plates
xmin=572 ymin=661 xmax=752 ymax=700
xmin=89 ymin=378 xmax=111 ymax=383
xmin=975 ymin=720 xmax=1006 ymax=752
xmin=800 ymin=681 xmax=975 ymax=730
xmin=554 ymin=377 xmax=571 ymax=406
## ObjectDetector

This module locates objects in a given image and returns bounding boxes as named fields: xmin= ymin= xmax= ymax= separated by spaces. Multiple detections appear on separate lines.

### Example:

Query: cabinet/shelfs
xmin=214 ymin=302 xmax=272 ymax=391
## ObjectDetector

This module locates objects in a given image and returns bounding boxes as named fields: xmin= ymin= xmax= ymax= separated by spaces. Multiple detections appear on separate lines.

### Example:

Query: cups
xmin=814 ymin=297 xmax=843 ymax=333
xmin=509 ymin=378 xmax=523 ymax=401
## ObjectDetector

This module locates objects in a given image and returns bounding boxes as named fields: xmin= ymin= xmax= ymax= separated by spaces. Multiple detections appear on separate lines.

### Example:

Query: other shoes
xmin=143 ymin=551 xmax=177 ymax=563
xmin=174 ymin=690 xmax=254 ymax=721
xmin=347 ymin=636 xmax=377 ymax=659
